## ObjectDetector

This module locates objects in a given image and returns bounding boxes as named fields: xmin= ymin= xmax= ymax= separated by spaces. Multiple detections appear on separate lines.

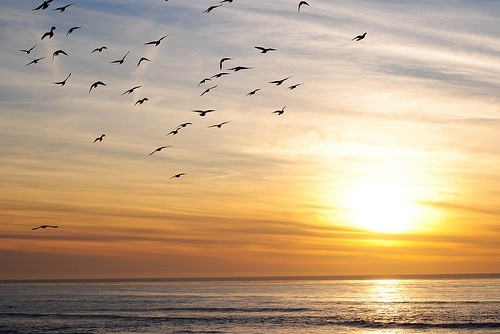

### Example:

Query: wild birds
xmin=269 ymin=76 xmax=293 ymax=86
xmin=287 ymin=83 xmax=302 ymax=90
xmin=150 ymin=145 xmax=172 ymax=156
xmin=166 ymin=128 xmax=182 ymax=136
xmin=209 ymin=120 xmax=230 ymax=128
xmin=135 ymin=98 xmax=149 ymax=105
xmin=200 ymin=85 xmax=217 ymax=96
xmin=32 ymin=225 xmax=58 ymax=230
xmin=272 ymin=106 xmax=286 ymax=115
xmin=255 ymin=46 xmax=276 ymax=53
xmin=177 ymin=123 xmax=192 ymax=128
xmin=228 ymin=66 xmax=253 ymax=71
xmin=197 ymin=78 xmax=211 ymax=87
xmin=193 ymin=110 xmax=214 ymax=116
xmin=18 ymin=0 xmax=310 ymax=96
xmin=352 ymin=32 xmax=367 ymax=41
xmin=247 ymin=88 xmax=261 ymax=96
xmin=93 ymin=134 xmax=106 ymax=143
xmin=211 ymin=73 xmax=229 ymax=79
xmin=170 ymin=172 xmax=186 ymax=179
